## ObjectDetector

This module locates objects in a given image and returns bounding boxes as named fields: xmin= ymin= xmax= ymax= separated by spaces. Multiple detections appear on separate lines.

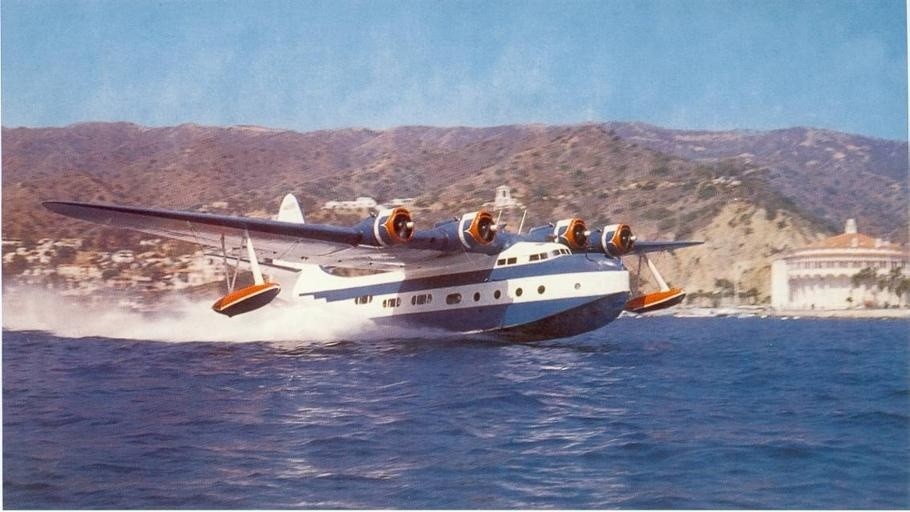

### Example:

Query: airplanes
xmin=39 ymin=184 xmax=710 ymax=345
